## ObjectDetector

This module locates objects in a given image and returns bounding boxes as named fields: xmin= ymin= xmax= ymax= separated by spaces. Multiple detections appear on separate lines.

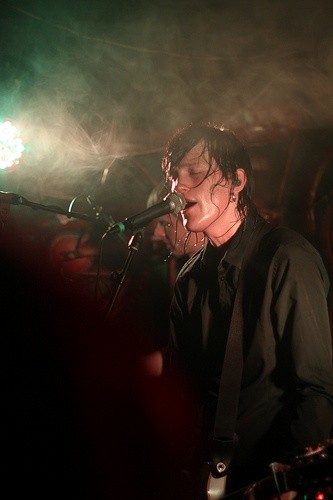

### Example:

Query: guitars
xmin=187 ymin=431 xmax=333 ymax=500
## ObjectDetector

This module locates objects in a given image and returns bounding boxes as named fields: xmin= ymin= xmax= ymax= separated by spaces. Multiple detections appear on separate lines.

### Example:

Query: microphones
xmin=105 ymin=191 xmax=186 ymax=236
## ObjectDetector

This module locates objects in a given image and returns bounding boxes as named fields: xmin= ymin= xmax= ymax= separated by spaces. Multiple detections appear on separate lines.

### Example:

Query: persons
xmin=162 ymin=125 xmax=333 ymax=500
xmin=146 ymin=178 xmax=208 ymax=260
